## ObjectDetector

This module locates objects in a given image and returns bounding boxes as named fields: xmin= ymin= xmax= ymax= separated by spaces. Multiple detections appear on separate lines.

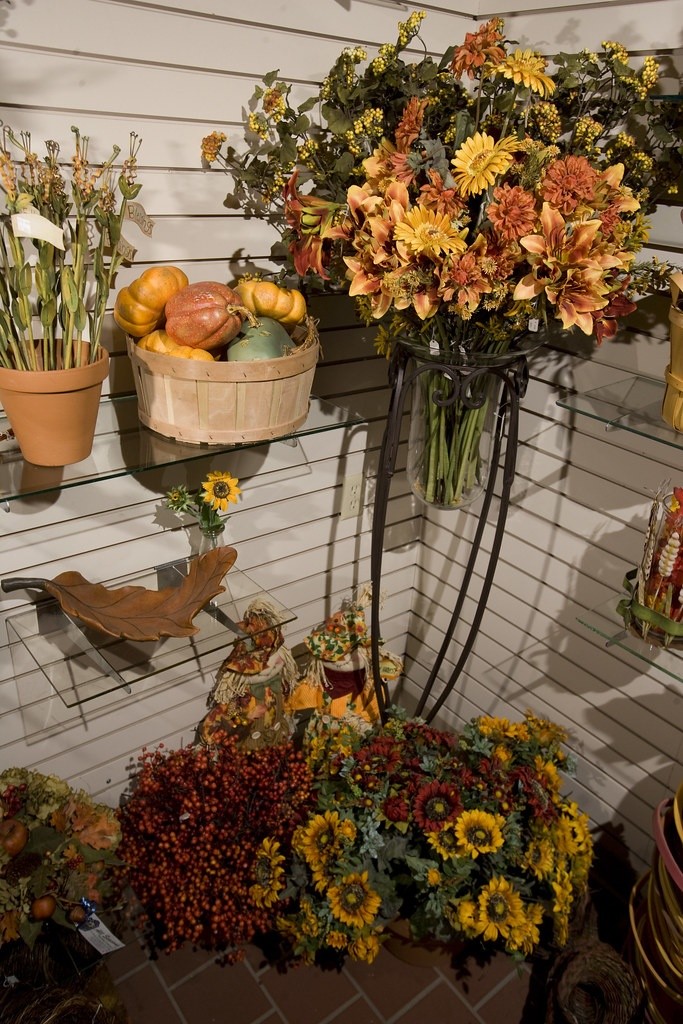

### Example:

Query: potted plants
xmin=0 ymin=338 xmax=110 ymax=466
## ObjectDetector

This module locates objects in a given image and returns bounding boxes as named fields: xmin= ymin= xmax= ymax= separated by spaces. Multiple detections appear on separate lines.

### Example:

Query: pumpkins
xmin=112 ymin=266 xmax=310 ymax=362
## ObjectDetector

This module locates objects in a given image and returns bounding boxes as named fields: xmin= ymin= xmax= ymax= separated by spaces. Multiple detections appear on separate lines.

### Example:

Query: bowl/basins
xmin=126 ymin=331 xmax=319 ymax=444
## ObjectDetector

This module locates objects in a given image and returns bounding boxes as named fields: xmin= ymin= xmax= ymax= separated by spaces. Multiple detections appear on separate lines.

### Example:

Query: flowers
xmin=0 ymin=766 xmax=123 ymax=953
xmin=167 ymin=470 xmax=242 ymax=551
xmin=0 ymin=120 xmax=154 ymax=375
xmin=203 ymin=12 xmax=683 ymax=502
xmin=248 ymin=710 xmax=598 ymax=970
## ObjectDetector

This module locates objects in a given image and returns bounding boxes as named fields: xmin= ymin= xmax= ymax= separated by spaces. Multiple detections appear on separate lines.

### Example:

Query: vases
xmin=398 ymin=334 xmax=501 ymax=510
xmin=196 ymin=526 xmax=226 ymax=558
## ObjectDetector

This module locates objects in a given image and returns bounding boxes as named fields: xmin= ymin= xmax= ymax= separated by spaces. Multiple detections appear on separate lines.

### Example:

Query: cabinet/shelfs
xmin=555 ymin=375 xmax=683 ymax=684
xmin=0 ymin=391 xmax=366 ymax=711
